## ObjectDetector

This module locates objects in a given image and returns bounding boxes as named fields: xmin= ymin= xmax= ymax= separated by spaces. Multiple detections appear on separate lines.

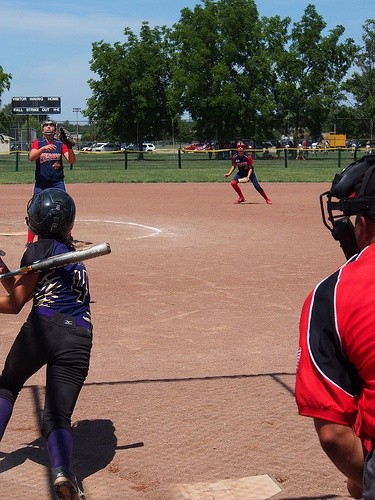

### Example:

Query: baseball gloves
xmin=58 ymin=126 xmax=76 ymax=148
xmin=238 ymin=177 xmax=249 ymax=183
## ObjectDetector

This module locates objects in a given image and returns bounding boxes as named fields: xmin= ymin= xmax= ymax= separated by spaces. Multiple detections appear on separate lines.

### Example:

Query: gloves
xmin=238 ymin=177 xmax=249 ymax=183
xmin=58 ymin=129 xmax=76 ymax=149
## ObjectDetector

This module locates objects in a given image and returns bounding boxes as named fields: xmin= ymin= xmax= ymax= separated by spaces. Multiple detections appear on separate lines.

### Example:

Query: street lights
xmin=72 ymin=108 xmax=82 ymax=142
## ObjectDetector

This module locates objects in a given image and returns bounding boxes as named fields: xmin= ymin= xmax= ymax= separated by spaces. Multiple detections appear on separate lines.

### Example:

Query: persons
xmin=345 ymin=142 xmax=370 ymax=153
xmin=25 ymin=120 xmax=75 ymax=245
xmin=0 ymin=188 xmax=92 ymax=500
xmin=207 ymin=145 xmax=212 ymax=158
xmin=223 ymin=140 xmax=273 ymax=204
xmin=277 ymin=139 xmax=321 ymax=161
xmin=224 ymin=142 xmax=237 ymax=158
xmin=294 ymin=154 xmax=375 ymax=500
xmin=324 ymin=141 xmax=330 ymax=148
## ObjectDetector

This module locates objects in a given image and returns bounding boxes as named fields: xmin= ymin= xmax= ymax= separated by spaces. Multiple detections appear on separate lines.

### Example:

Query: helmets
xmin=237 ymin=147 xmax=243 ymax=152
xmin=26 ymin=188 xmax=76 ymax=239
xmin=332 ymin=152 xmax=375 ymax=222
xmin=40 ymin=119 xmax=57 ymax=134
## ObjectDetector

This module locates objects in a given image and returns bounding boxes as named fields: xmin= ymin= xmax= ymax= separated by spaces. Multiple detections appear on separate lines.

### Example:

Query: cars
xmin=344 ymin=138 xmax=361 ymax=148
xmin=82 ymin=142 xmax=119 ymax=152
xmin=182 ymin=142 xmax=216 ymax=154
xmin=242 ymin=138 xmax=330 ymax=152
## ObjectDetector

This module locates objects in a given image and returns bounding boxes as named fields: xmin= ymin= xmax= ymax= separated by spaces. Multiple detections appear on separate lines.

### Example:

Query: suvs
xmin=120 ymin=142 xmax=155 ymax=153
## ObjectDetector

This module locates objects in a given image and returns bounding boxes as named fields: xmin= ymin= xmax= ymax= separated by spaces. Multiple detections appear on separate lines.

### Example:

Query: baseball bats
xmin=0 ymin=243 xmax=112 ymax=280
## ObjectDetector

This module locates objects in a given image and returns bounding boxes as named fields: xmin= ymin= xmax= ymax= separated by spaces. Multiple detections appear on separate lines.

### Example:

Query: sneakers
xmin=51 ymin=467 xmax=86 ymax=500
xmin=233 ymin=198 xmax=246 ymax=204
xmin=266 ymin=198 xmax=272 ymax=204
